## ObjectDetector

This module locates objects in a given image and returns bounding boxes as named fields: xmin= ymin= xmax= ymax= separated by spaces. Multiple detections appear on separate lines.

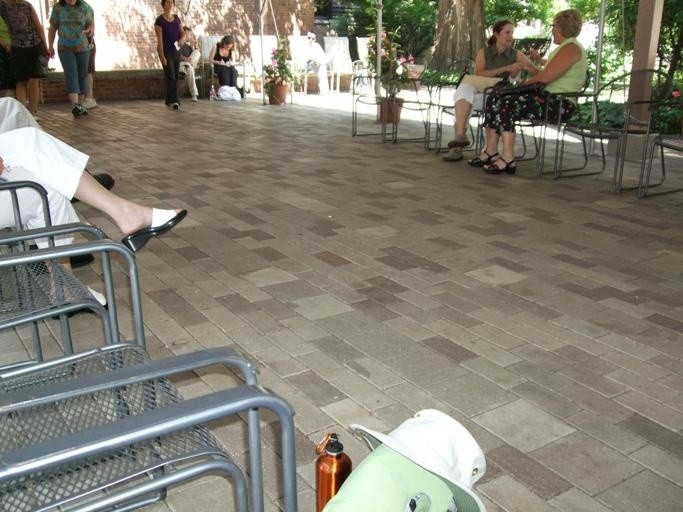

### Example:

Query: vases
xmin=264 ymin=84 xmax=289 ymax=106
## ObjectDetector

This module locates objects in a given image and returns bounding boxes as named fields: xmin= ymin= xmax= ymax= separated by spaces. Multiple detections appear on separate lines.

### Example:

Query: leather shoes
xmin=30 ymin=245 xmax=94 ymax=276
xmin=70 ymin=174 xmax=113 ymax=203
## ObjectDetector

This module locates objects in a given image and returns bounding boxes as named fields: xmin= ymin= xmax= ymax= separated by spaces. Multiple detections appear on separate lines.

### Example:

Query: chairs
xmin=198 ymin=35 xmax=391 ymax=101
xmin=348 ymin=54 xmax=682 ymax=199
xmin=0 ymin=175 xmax=299 ymax=512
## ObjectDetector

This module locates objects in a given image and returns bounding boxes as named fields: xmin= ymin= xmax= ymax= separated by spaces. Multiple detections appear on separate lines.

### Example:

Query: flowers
xmin=367 ymin=26 xmax=415 ymax=95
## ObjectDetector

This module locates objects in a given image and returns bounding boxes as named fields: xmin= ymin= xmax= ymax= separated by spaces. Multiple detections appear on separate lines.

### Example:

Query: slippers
xmin=51 ymin=288 xmax=107 ymax=319
xmin=122 ymin=209 xmax=185 ymax=253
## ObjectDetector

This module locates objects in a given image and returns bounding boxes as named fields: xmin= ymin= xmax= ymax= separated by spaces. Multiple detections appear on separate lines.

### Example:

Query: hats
xmin=350 ymin=409 xmax=486 ymax=512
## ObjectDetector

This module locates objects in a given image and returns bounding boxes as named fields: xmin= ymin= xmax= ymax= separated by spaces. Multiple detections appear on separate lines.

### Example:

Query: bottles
xmin=210 ymin=85 xmax=216 ymax=100
xmin=316 ymin=432 xmax=354 ymax=511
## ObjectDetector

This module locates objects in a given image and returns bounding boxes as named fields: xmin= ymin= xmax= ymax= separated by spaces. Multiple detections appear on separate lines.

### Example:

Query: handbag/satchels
xmin=459 ymin=71 xmax=503 ymax=95
xmin=502 ymin=83 xmax=542 ymax=94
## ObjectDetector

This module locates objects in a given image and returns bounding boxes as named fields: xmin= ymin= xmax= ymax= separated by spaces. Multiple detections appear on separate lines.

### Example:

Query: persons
xmin=4 ymin=0 xmax=52 ymax=115
xmin=0 ymin=126 xmax=188 ymax=321
xmin=0 ymin=1 xmax=17 ymax=99
xmin=439 ymin=19 xmax=540 ymax=162
xmin=0 ymin=95 xmax=115 ymax=203
xmin=465 ymin=8 xmax=587 ymax=175
xmin=175 ymin=25 xmax=202 ymax=102
xmin=152 ymin=1 xmax=187 ymax=110
xmin=43 ymin=0 xmax=94 ymax=118
xmin=207 ymin=35 xmax=238 ymax=87
xmin=83 ymin=37 xmax=98 ymax=109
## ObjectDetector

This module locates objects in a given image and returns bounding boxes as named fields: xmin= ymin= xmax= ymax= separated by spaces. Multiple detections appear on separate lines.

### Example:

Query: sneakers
xmin=443 ymin=148 xmax=463 ymax=162
xmin=447 ymin=138 xmax=470 ymax=148
xmin=72 ymin=99 xmax=97 ymax=117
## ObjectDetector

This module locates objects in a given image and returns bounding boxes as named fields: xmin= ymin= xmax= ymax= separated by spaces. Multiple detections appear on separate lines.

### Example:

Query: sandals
xmin=468 ymin=152 xmax=499 ymax=167
xmin=484 ymin=157 xmax=516 ymax=174
